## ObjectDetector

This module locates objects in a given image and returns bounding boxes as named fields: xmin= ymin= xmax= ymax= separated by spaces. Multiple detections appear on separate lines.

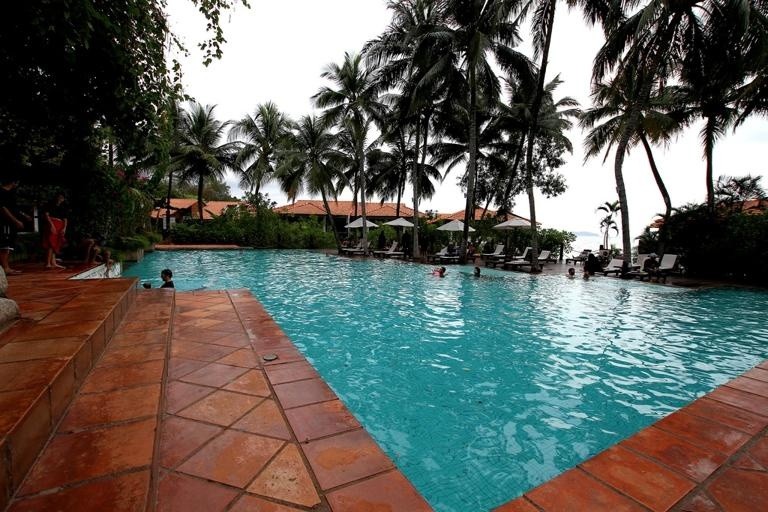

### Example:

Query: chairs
xmin=569 ymin=243 xmax=686 ymax=283
xmin=340 ymin=238 xmax=557 ymax=272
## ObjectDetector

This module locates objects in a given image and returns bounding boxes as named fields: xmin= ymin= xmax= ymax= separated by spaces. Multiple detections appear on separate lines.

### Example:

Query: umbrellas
xmin=491 ymin=216 xmax=539 ymax=233
xmin=435 ymin=218 xmax=477 ymax=233
xmin=382 ymin=216 xmax=421 ymax=233
xmin=343 ymin=217 xmax=379 ymax=230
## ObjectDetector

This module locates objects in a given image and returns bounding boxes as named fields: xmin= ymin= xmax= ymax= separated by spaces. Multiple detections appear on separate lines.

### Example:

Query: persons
xmin=43 ymin=190 xmax=69 ymax=269
xmin=1 ymin=170 xmax=31 ymax=275
xmin=159 ymin=269 xmax=174 ymax=288
xmin=65 ymin=232 xmax=114 ymax=271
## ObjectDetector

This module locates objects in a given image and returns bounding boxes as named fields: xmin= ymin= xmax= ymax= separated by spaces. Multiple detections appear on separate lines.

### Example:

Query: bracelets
xmin=22 ymin=212 xmax=25 ymax=216
xmin=14 ymin=218 xmax=17 ymax=223
